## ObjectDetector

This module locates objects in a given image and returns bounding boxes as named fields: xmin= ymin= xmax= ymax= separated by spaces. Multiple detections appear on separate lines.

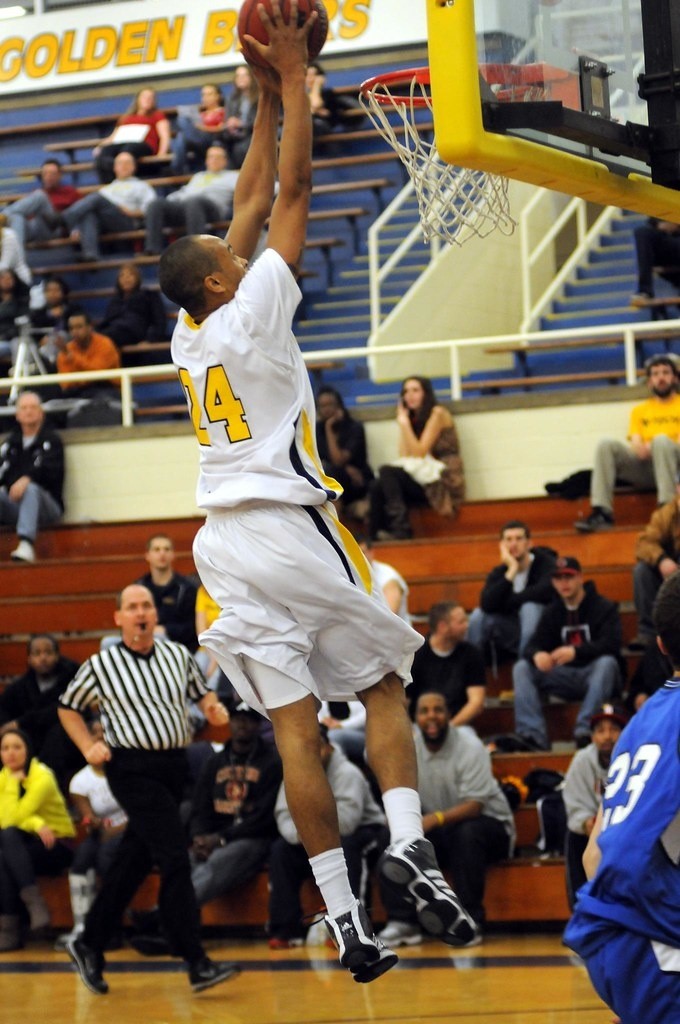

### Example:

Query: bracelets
xmin=435 ymin=810 xmax=444 ymax=825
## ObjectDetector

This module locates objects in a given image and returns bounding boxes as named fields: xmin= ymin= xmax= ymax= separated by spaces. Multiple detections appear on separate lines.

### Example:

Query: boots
xmin=0 ymin=914 xmax=21 ymax=951
xmin=20 ymin=885 xmax=49 ymax=929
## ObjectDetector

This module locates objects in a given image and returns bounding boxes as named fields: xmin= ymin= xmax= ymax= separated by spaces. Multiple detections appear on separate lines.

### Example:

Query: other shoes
xmin=40 ymin=210 xmax=59 ymax=233
xmin=532 ymin=851 xmax=565 ymax=867
xmin=54 ymin=923 xmax=87 ymax=951
xmin=377 ymin=919 xmax=424 ymax=948
xmin=575 ymin=506 xmax=617 ymax=534
xmin=494 ymin=733 xmax=544 ymax=754
xmin=127 ymin=905 xmax=175 ymax=956
xmin=75 ymin=251 xmax=97 ymax=263
xmin=379 ymin=522 xmax=414 ymax=543
xmin=627 ymin=636 xmax=652 ymax=654
xmin=268 ymin=935 xmax=303 ymax=949
xmin=10 ymin=539 xmax=35 ymax=562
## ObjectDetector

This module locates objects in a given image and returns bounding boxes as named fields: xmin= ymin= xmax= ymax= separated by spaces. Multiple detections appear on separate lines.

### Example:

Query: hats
xmin=547 ymin=556 xmax=581 ymax=578
xmin=589 ymin=702 xmax=627 ymax=731
xmin=229 ymin=699 xmax=264 ymax=724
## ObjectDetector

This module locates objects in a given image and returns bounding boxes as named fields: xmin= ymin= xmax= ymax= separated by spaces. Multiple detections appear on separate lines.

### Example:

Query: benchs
xmin=0 ymin=487 xmax=658 ymax=923
xmin=0 ymin=58 xmax=436 ymax=419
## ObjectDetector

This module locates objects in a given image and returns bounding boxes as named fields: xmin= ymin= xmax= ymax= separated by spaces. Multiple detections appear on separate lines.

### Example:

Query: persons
xmin=316 ymin=386 xmax=373 ymax=518
xmin=367 ymin=375 xmax=466 ymax=540
xmin=1 ymin=534 xmax=387 ymax=948
xmin=562 ymin=569 xmax=680 ymax=1024
xmin=58 ymin=583 xmax=239 ymax=996
xmin=631 ymin=215 xmax=680 ymax=300
xmin=574 ymin=356 xmax=680 ymax=532
xmin=170 ymin=1 xmax=480 ymax=983
xmin=0 ymin=392 xmax=64 ymax=562
xmin=354 ymin=479 xmax=680 ymax=950
xmin=0 ymin=64 xmax=341 ymax=282
xmin=0 ymin=223 xmax=169 ymax=427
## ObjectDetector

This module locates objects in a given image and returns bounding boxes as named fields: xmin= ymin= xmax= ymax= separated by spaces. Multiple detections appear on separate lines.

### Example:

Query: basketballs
xmin=237 ymin=0 xmax=330 ymax=70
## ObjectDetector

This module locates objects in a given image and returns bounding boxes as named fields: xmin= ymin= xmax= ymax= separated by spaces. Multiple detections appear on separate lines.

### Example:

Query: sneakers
xmin=68 ymin=939 xmax=109 ymax=994
xmin=323 ymin=898 xmax=398 ymax=983
xmin=378 ymin=835 xmax=478 ymax=946
xmin=188 ymin=961 xmax=238 ymax=992
